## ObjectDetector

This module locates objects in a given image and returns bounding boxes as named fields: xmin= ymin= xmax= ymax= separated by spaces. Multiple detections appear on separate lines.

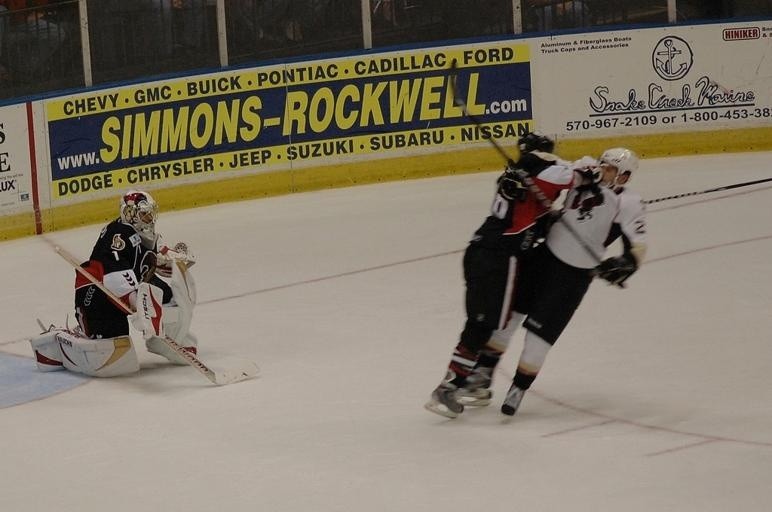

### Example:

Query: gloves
xmin=594 ymin=258 xmax=636 ymax=284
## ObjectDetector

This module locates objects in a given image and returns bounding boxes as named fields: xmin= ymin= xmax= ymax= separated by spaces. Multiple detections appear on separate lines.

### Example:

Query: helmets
xmin=121 ymin=191 xmax=157 ymax=250
xmin=517 ymin=133 xmax=554 ymax=160
xmin=599 ymin=147 xmax=637 ymax=190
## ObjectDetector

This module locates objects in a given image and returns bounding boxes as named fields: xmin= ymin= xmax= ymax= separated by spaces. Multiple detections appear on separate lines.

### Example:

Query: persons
xmin=447 ymin=145 xmax=650 ymax=417
xmin=71 ymin=188 xmax=202 ymax=368
xmin=430 ymin=132 xmax=601 ymax=421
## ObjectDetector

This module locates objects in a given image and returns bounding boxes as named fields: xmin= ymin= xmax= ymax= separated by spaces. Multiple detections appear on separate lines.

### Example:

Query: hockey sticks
xmin=450 ymin=57 xmax=624 ymax=290
xmin=55 ymin=245 xmax=262 ymax=386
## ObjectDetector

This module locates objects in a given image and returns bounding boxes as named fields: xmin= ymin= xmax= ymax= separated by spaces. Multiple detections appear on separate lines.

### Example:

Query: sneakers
xmin=502 ymin=383 xmax=525 ymax=416
xmin=431 ymin=384 xmax=464 ymax=414
xmin=461 ymin=369 xmax=491 ymax=389
